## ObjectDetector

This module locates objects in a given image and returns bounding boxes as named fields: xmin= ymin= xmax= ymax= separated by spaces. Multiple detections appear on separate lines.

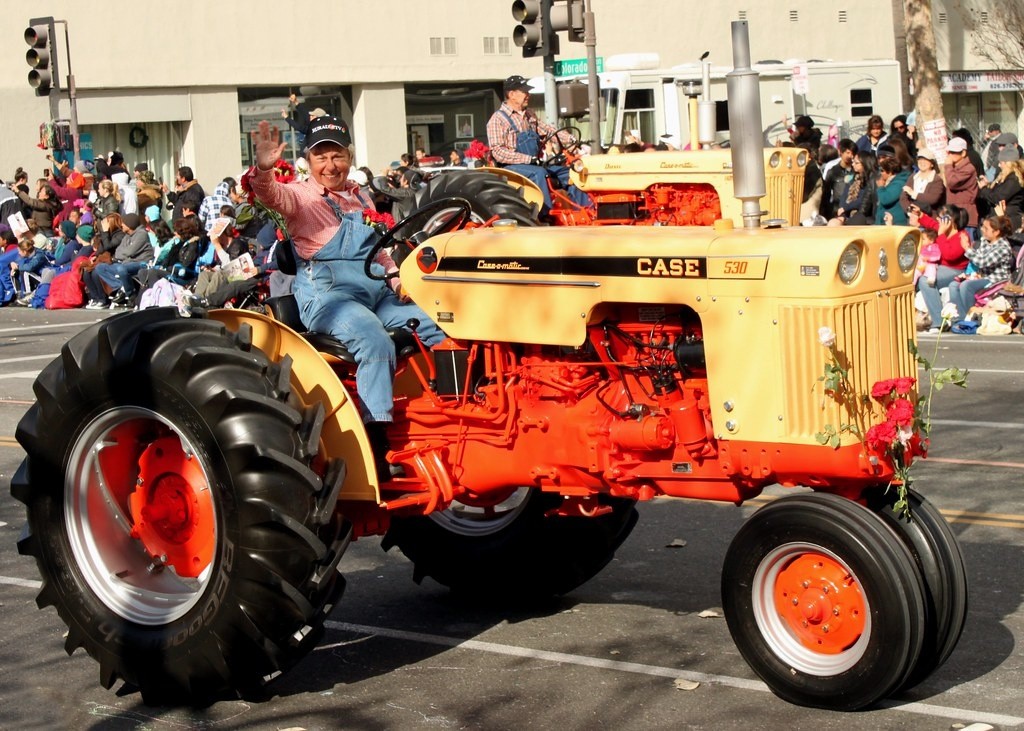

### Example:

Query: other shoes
xmin=366 ymin=421 xmax=405 ymax=478
xmin=17 ymin=297 xmax=32 ymax=305
xmin=86 ymin=298 xmax=107 ymax=309
xmin=928 ymin=323 xmax=950 ymax=332
xmin=112 ymin=292 xmax=136 ymax=303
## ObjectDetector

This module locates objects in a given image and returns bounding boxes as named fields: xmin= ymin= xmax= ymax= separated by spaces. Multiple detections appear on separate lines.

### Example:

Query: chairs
xmin=110 ymin=238 xmax=263 ymax=311
xmin=488 ymin=150 xmax=564 ymax=191
xmin=264 ymin=239 xmax=429 ymax=365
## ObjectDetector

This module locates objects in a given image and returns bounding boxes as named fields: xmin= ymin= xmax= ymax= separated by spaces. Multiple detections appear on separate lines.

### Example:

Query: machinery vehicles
xmin=413 ymin=60 xmax=810 ymax=231
xmin=9 ymin=19 xmax=972 ymax=711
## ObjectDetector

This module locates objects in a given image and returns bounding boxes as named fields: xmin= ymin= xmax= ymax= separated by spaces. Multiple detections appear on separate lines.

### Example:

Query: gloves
xmin=531 ymin=155 xmax=540 ymax=166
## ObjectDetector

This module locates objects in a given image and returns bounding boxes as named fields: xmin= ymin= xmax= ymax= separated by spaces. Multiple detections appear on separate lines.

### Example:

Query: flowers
xmin=811 ymin=302 xmax=970 ymax=516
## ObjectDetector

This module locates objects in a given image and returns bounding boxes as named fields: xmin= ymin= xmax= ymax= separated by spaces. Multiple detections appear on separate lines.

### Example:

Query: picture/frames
xmin=456 ymin=113 xmax=474 ymax=138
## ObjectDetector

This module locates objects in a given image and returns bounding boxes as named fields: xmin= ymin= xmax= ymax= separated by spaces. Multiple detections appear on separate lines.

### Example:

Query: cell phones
xmin=47 ymin=155 xmax=50 ymax=159
xmin=158 ymin=176 xmax=163 ymax=185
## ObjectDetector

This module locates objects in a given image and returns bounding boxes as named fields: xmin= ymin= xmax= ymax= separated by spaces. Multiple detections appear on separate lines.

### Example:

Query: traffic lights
xmin=39 ymin=123 xmax=71 ymax=150
xmin=549 ymin=0 xmax=584 ymax=43
xmin=511 ymin=0 xmax=544 ymax=57
xmin=24 ymin=15 xmax=61 ymax=97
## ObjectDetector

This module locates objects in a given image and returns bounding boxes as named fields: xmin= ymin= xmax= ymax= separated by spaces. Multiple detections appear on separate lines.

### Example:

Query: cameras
xmin=907 ymin=206 xmax=913 ymax=212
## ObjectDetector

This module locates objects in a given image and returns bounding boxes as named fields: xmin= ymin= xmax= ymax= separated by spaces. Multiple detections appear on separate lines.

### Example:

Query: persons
xmin=486 ymin=76 xmax=595 ymax=217
xmin=345 ymin=147 xmax=486 ymax=223
xmin=606 ymin=131 xmax=721 ymax=153
xmin=773 ymin=114 xmax=1024 ymax=335
xmin=246 ymin=116 xmax=449 ymax=482
xmin=0 ymin=150 xmax=309 ymax=309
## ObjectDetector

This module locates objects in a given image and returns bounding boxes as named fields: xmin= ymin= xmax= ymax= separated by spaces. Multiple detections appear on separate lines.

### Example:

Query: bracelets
xmin=56 ymin=163 xmax=59 ymax=166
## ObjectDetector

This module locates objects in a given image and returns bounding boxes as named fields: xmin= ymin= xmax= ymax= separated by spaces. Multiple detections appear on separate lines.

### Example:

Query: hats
xmin=121 ymin=214 xmax=140 ymax=232
xmin=73 ymin=159 xmax=95 ymax=174
xmin=145 ymin=205 xmax=160 ymax=222
xmin=69 ymin=173 xmax=78 ymax=182
xmin=306 ymin=117 xmax=352 ymax=151
xmin=504 ymin=76 xmax=534 ymax=94
xmin=308 ymin=108 xmax=325 ymax=119
xmin=60 ymin=220 xmax=75 ymax=238
xmin=135 ymin=162 xmax=147 ymax=173
xmin=346 ymin=169 xmax=367 ymax=187
xmin=77 ymin=225 xmax=94 ymax=242
xmin=917 ymin=124 xmax=1019 ymax=161
xmin=792 ymin=116 xmax=814 ymax=128
xmin=403 ymin=170 xmax=420 ymax=192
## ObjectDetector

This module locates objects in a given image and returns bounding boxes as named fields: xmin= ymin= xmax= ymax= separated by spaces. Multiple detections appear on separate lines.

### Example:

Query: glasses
xmin=895 ymin=125 xmax=905 ymax=131
xmin=851 ymin=158 xmax=862 ymax=165
